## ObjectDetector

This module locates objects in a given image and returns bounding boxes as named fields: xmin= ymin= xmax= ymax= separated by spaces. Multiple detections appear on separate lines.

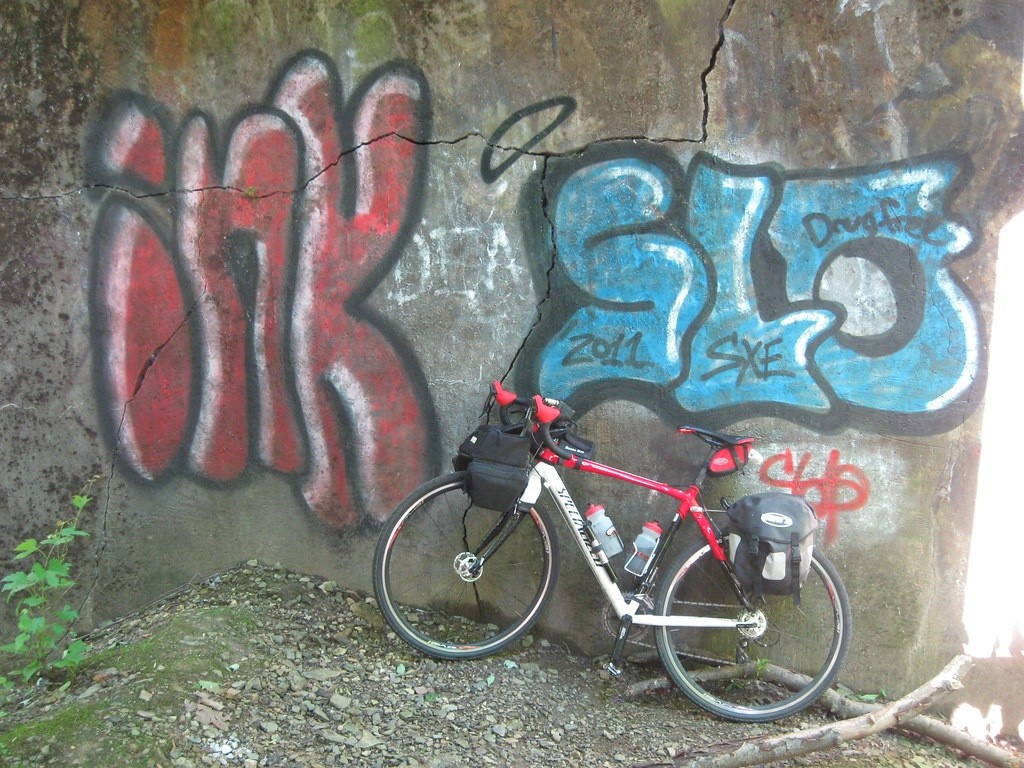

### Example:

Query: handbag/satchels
xmin=726 ymin=491 xmax=820 ymax=607
xmin=452 ymin=423 xmax=529 ymax=512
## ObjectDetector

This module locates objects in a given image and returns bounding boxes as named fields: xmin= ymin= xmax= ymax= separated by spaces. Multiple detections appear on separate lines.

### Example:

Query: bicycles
xmin=371 ymin=380 xmax=853 ymax=723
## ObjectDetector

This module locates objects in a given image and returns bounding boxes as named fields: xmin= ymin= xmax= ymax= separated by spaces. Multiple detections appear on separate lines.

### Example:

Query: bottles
xmin=585 ymin=501 xmax=623 ymax=558
xmin=625 ymin=520 xmax=661 ymax=575
